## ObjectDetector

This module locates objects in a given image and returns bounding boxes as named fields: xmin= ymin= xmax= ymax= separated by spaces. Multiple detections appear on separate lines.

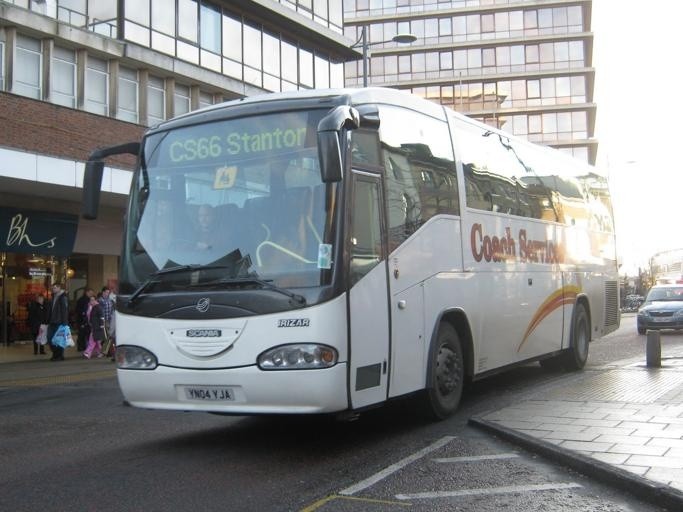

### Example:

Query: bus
xmin=83 ymin=86 xmax=620 ymax=423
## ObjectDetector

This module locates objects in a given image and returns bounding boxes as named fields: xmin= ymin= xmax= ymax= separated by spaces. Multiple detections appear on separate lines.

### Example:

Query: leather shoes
xmin=51 ymin=351 xmax=64 ymax=362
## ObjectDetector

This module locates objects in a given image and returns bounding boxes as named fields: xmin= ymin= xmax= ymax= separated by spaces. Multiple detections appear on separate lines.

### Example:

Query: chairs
xmin=213 ymin=184 xmax=325 ymax=247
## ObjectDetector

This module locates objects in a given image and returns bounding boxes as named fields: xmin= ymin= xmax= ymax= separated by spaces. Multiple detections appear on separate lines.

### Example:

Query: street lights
xmin=350 ymin=25 xmax=417 ymax=86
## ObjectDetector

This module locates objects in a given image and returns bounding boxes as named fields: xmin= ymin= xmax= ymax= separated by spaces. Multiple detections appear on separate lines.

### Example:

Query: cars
xmin=637 ymin=284 xmax=683 ymax=335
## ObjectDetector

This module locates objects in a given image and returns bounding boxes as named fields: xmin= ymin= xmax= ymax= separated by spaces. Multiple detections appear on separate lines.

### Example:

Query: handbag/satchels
xmin=35 ymin=324 xmax=49 ymax=346
xmin=100 ymin=336 xmax=115 ymax=357
xmin=51 ymin=325 xmax=75 ymax=349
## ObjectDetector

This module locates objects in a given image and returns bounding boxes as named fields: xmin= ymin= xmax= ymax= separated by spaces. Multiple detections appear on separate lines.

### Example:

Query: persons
xmin=78 ymin=277 xmax=117 ymax=362
xmin=195 ymin=204 xmax=217 ymax=250
xmin=47 ymin=282 xmax=70 ymax=362
xmin=27 ymin=293 xmax=48 ymax=354
